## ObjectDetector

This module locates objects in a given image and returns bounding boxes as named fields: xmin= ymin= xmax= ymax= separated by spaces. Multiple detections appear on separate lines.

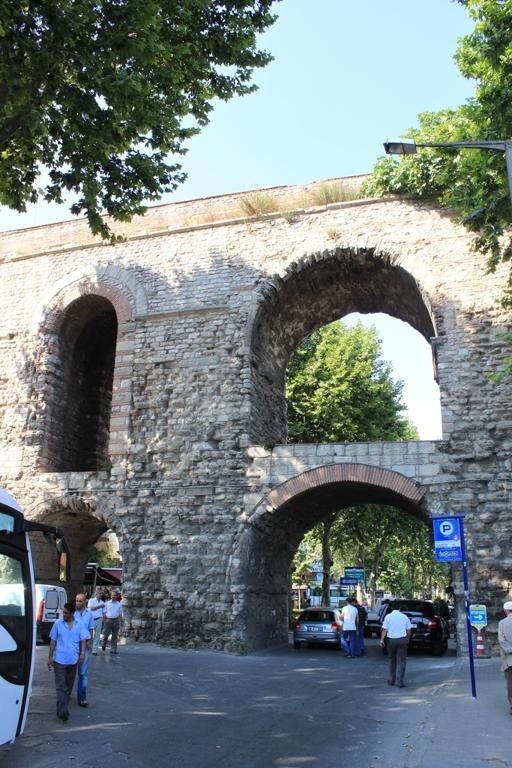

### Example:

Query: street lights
xmin=382 ymin=136 xmax=512 ymax=201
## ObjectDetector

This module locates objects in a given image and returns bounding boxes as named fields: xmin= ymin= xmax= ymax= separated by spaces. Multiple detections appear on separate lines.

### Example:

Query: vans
xmin=1 ymin=582 xmax=68 ymax=646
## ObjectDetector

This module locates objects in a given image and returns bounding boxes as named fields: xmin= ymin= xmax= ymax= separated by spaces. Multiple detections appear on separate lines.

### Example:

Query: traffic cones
xmin=473 ymin=632 xmax=491 ymax=659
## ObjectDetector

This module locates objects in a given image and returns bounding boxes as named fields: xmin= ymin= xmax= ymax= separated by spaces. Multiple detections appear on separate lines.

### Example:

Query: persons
xmin=46 ymin=586 xmax=126 ymax=722
xmin=337 ymin=596 xmax=413 ymax=688
xmin=497 ymin=600 xmax=512 ymax=715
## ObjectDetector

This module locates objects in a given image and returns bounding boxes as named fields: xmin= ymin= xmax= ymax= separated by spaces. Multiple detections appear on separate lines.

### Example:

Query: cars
xmin=293 ymin=608 xmax=341 ymax=650
xmin=364 ymin=599 xmax=457 ymax=658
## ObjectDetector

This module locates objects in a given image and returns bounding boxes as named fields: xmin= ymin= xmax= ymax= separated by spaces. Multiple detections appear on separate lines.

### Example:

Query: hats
xmin=502 ymin=599 xmax=512 ymax=610
xmin=63 ymin=603 xmax=76 ymax=613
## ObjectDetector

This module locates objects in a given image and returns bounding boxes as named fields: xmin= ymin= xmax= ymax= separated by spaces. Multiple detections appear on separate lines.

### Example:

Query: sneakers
xmin=56 ymin=697 xmax=92 ymax=722
xmin=387 ymin=679 xmax=407 ymax=688
xmin=92 ymin=644 xmax=121 ymax=656
xmin=344 ymin=649 xmax=368 ymax=658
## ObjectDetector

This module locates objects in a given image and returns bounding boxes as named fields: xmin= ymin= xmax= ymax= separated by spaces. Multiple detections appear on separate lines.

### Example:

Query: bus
xmin=383 ymin=591 xmax=392 ymax=598
xmin=308 ymin=585 xmax=383 ymax=610
xmin=1 ymin=488 xmax=72 ymax=749
